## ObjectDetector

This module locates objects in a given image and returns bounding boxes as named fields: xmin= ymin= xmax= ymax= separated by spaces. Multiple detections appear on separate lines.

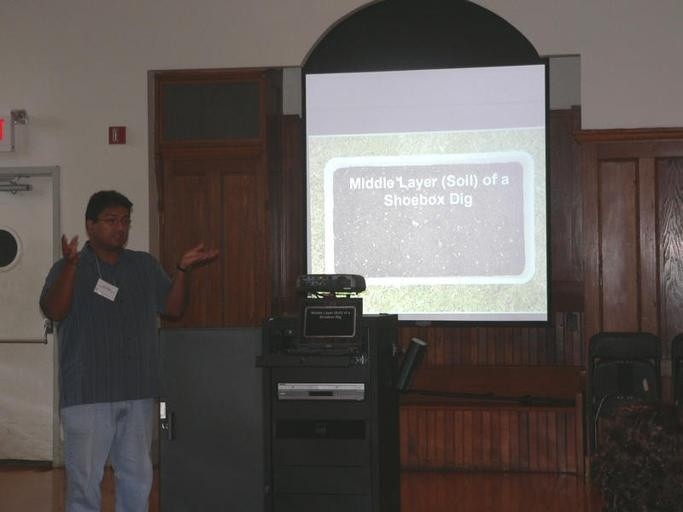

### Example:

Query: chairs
xmin=588 ymin=332 xmax=659 ymax=443
xmin=671 ymin=334 xmax=682 ymax=393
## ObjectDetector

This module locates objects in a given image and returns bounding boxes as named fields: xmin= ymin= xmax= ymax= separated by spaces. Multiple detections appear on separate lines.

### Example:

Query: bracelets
xmin=176 ymin=264 xmax=189 ymax=273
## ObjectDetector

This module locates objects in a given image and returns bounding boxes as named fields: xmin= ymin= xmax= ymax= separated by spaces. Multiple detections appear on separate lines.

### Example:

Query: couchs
xmin=600 ymin=395 xmax=682 ymax=512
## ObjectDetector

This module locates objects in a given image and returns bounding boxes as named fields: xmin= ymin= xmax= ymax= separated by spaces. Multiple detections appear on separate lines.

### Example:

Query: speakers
xmin=548 ymin=57 xmax=573 ymax=110
xmin=281 ymin=67 xmax=304 ymax=119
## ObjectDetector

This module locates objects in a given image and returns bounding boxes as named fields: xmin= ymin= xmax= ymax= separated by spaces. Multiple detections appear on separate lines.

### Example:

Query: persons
xmin=40 ymin=190 xmax=221 ymax=512
